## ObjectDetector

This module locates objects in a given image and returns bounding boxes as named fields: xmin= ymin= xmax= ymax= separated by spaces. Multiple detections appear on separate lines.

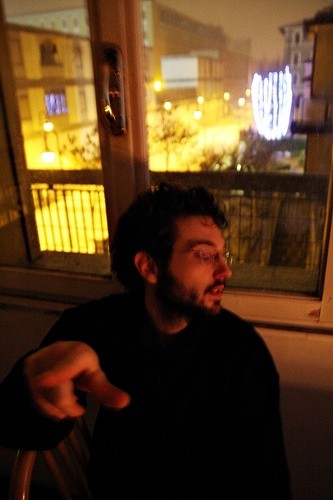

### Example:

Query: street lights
xmin=43 ymin=122 xmax=54 ymax=154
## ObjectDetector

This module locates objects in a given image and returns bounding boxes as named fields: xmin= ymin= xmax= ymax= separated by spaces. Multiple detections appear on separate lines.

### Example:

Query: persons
xmin=0 ymin=181 xmax=294 ymax=500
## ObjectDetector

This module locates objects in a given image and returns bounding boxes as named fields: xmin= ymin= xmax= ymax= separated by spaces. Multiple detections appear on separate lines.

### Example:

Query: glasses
xmin=170 ymin=242 xmax=237 ymax=270
